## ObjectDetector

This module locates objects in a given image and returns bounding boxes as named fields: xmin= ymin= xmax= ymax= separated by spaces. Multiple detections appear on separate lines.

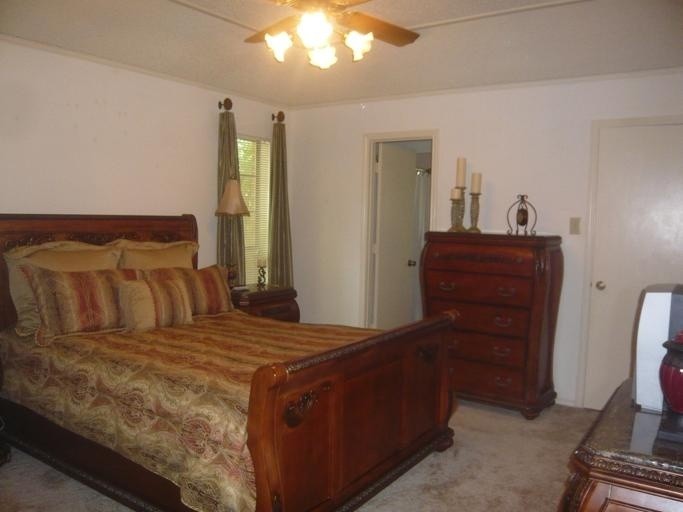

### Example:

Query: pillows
xmin=18 ymin=262 xmax=146 ymax=347
xmin=108 ymin=239 xmax=199 ymax=330
xmin=144 ymin=263 xmax=235 ymax=317
xmin=113 ymin=277 xmax=194 ymax=333
xmin=1 ymin=239 xmax=127 ymax=339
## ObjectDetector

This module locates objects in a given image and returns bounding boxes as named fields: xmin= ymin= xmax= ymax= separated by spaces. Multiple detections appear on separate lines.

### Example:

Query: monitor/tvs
xmin=629 ymin=284 xmax=683 ymax=416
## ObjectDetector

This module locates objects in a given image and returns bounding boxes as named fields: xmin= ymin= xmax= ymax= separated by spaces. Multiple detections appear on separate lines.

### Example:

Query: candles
xmin=258 ymin=259 xmax=266 ymax=267
xmin=470 ymin=173 xmax=481 ymax=194
xmin=451 ymin=189 xmax=461 ymax=199
xmin=456 ymin=158 xmax=466 ymax=187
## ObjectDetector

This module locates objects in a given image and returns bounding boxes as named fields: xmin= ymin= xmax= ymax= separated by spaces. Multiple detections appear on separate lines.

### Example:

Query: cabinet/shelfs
xmin=419 ymin=232 xmax=564 ymax=421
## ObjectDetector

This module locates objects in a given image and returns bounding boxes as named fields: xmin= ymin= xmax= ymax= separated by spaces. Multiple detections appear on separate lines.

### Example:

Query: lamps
xmin=214 ymin=175 xmax=250 ymax=292
xmin=258 ymin=9 xmax=375 ymax=73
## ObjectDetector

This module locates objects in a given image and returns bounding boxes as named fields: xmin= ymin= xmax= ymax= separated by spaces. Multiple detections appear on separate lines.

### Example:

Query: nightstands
xmin=230 ymin=286 xmax=301 ymax=324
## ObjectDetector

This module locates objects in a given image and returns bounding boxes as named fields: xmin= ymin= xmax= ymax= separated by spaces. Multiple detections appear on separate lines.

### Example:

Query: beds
xmin=0 ymin=214 xmax=465 ymax=511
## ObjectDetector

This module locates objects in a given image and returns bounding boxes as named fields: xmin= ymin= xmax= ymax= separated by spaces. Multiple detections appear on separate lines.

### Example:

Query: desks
xmin=560 ymin=378 xmax=682 ymax=510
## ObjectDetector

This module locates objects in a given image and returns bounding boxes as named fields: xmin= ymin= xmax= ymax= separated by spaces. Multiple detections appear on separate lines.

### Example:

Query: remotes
xmin=233 ymin=286 xmax=249 ymax=290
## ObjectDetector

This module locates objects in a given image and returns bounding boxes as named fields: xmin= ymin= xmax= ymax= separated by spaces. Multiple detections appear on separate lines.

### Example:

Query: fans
xmin=243 ymin=1 xmax=422 ymax=69
